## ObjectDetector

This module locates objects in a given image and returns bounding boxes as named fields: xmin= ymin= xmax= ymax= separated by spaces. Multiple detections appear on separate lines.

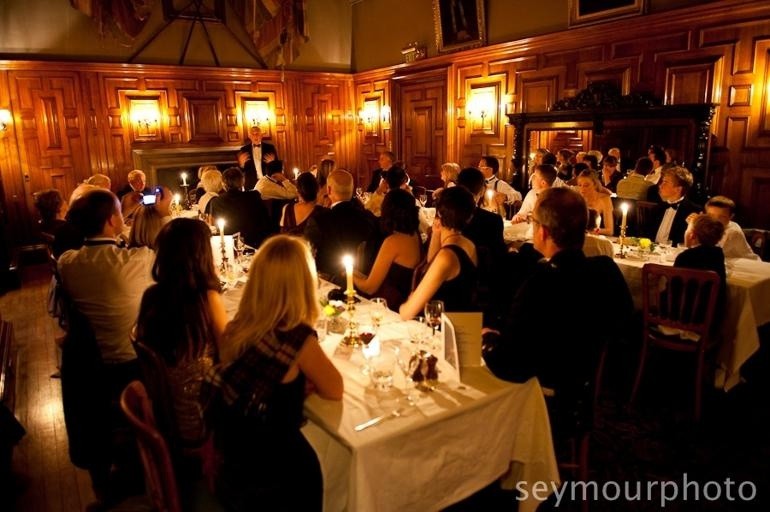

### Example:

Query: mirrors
xmin=523 ymin=120 xmax=696 ymax=189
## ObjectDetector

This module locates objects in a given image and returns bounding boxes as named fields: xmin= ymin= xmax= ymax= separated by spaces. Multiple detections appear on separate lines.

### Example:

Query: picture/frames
xmin=433 ymin=1 xmax=486 ymax=53
xmin=568 ymin=0 xmax=646 ymax=28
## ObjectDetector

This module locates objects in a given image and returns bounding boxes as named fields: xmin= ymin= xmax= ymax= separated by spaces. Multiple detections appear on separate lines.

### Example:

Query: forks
xmin=354 ymin=407 xmax=405 ymax=432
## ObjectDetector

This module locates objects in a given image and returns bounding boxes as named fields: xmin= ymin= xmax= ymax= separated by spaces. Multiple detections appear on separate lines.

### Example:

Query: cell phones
xmin=143 ymin=187 xmax=165 ymax=204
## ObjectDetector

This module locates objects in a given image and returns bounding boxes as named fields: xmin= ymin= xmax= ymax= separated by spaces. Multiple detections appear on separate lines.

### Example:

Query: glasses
xmin=526 ymin=211 xmax=540 ymax=224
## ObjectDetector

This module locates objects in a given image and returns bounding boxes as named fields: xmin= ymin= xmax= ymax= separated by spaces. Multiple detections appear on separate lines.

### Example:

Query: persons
xmin=34 ymin=123 xmax=756 ymax=512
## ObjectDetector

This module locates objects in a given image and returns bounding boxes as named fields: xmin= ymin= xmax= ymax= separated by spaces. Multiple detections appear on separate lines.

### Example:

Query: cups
xmin=314 ymin=315 xmax=327 ymax=343
xmin=659 ymin=240 xmax=673 ymax=262
xmin=225 ymin=258 xmax=240 ymax=282
xmin=407 ymin=316 xmax=428 ymax=345
xmin=369 ymin=297 xmax=388 ymax=320
xmin=370 ymin=356 xmax=394 ymax=390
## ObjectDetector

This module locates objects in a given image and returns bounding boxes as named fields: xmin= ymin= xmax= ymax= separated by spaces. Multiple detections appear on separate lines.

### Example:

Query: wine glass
xmin=420 ymin=195 xmax=427 ymax=208
xmin=395 ymin=345 xmax=420 ymax=405
xmin=425 ymin=300 xmax=444 ymax=352
xmin=233 ymin=237 xmax=245 ymax=262
xmin=356 ymin=187 xmax=363 ymax=197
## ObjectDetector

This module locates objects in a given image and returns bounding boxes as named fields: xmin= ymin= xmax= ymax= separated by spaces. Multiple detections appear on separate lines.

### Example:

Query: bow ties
xmin=253 ymin=144 xmax=261 ymax=148
xmin=664 ymin=202 xmax=677 ymax=210
